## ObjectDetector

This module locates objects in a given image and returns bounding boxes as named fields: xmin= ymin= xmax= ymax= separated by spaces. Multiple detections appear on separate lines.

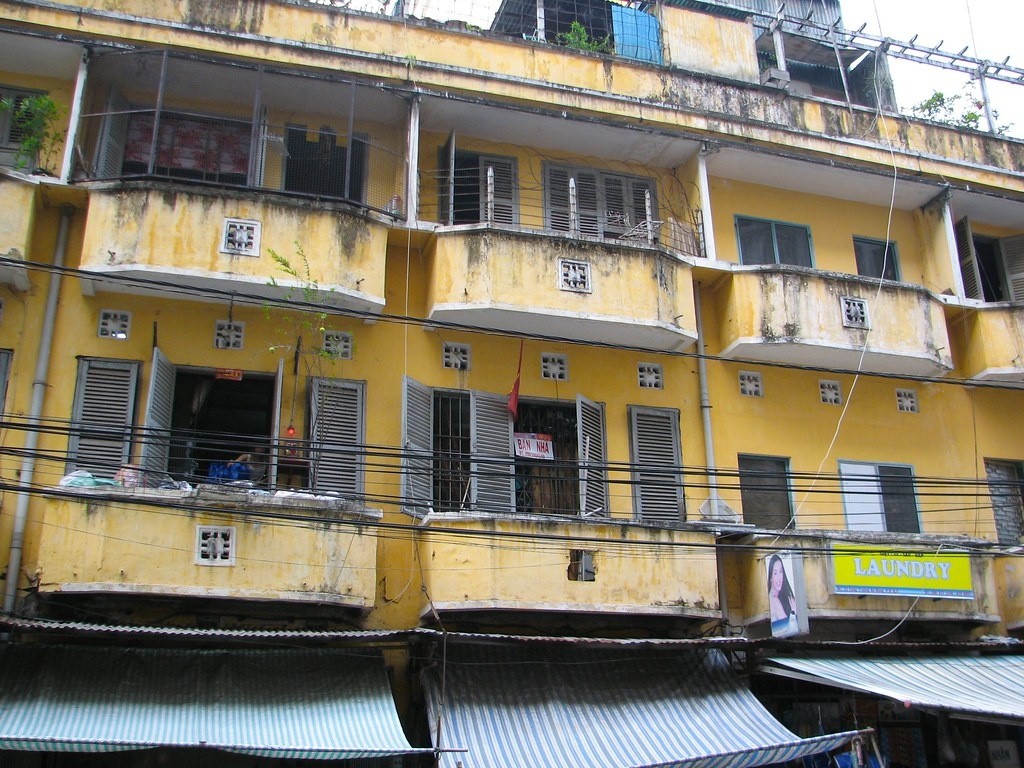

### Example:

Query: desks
xmin=278 ymin=456 xmax=313 ymax=489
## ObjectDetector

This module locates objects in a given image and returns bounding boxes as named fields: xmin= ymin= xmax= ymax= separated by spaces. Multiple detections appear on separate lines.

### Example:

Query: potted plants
xmin=0 ymin=96 xmax=68 ymax=176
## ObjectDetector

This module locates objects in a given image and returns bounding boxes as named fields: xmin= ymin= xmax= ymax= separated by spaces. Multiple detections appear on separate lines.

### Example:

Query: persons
xmin=228 ymin=443 xmax=270 ymax=486
xmin=766 ymin=554 xmax=797 ymax=623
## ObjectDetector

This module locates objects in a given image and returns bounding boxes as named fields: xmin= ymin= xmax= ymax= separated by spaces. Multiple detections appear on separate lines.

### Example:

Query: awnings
xmin=767 ymin=653 xmax=1024 ymax=718
xmin=0 ymin=640 xmax=469 ymax=761
xmin=418 ymin=655 xmax=874 ymax=768
